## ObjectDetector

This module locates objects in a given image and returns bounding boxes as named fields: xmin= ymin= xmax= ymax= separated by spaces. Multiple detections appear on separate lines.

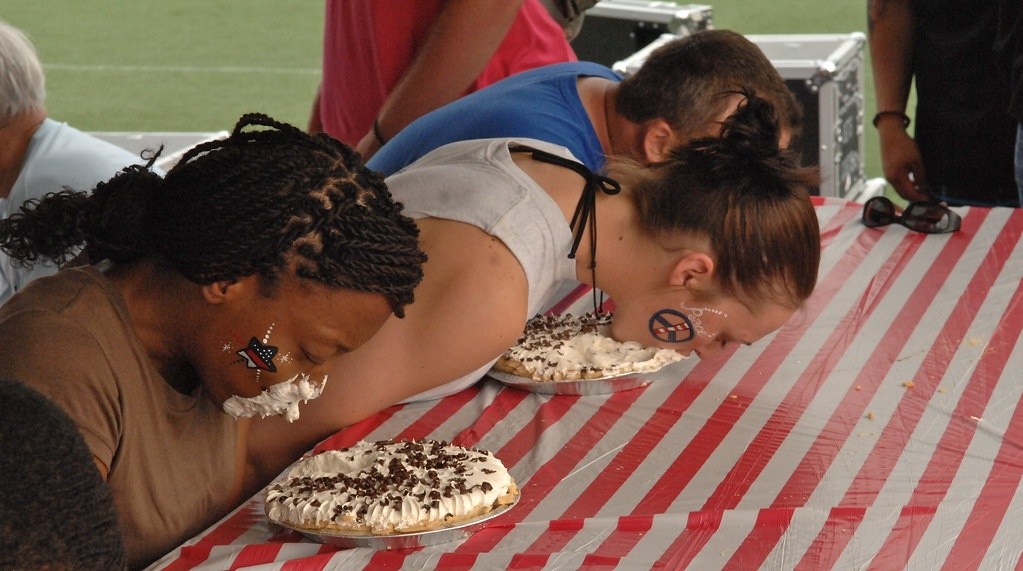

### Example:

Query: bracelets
xmin=872 ymin=110 xmax=911 ymax=131
xmin=373 ymin=117 xmax=386 ymax=147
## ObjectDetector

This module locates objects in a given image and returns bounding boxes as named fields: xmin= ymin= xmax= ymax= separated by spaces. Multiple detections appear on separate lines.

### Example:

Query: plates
xmin=488 ymin=370 xmax=662 ymax=395
xmin=265 ymin=486 xmax=521 ymax=548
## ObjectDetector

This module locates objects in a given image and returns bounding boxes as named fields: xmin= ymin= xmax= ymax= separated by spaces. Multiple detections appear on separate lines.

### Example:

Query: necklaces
xmin=588 ymin=179 xmax=604 ymax=317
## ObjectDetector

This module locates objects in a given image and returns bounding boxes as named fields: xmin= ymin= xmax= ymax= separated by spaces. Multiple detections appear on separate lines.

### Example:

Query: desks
xmin=148 ymin=193 xmax=1023 ymax=571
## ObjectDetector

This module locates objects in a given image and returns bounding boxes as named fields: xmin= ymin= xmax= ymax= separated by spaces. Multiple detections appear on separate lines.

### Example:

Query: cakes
xmin=496 ymin=307 xmax=678 ymax=379
xmin=269 ymin=439 xmax=516 ymax=530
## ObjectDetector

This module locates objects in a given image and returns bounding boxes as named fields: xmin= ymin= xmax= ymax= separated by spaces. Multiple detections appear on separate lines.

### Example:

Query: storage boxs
xmin=583 ymin=1 xmax=867 ymax=203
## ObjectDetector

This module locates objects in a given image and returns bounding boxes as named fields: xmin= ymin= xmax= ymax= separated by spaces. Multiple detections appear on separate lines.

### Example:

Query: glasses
xmin=862 ymin=196 xmax=961 ymax=234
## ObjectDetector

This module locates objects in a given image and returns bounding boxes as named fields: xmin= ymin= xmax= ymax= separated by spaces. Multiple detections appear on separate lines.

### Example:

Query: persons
xmin=244 ymin=83 xmax=822 ymax=486
xmin=865 ymin=0 xmax=1023 ymax=208
xmin=0 ymin=114 xmax=429 ymax=571
xmin=307 ymin=0 xmax=581 ymax=164
xmin=0 ymin=22 xmax=168 ymax=309
xmin=364 ymin=28 xmax=800 ymax=180
